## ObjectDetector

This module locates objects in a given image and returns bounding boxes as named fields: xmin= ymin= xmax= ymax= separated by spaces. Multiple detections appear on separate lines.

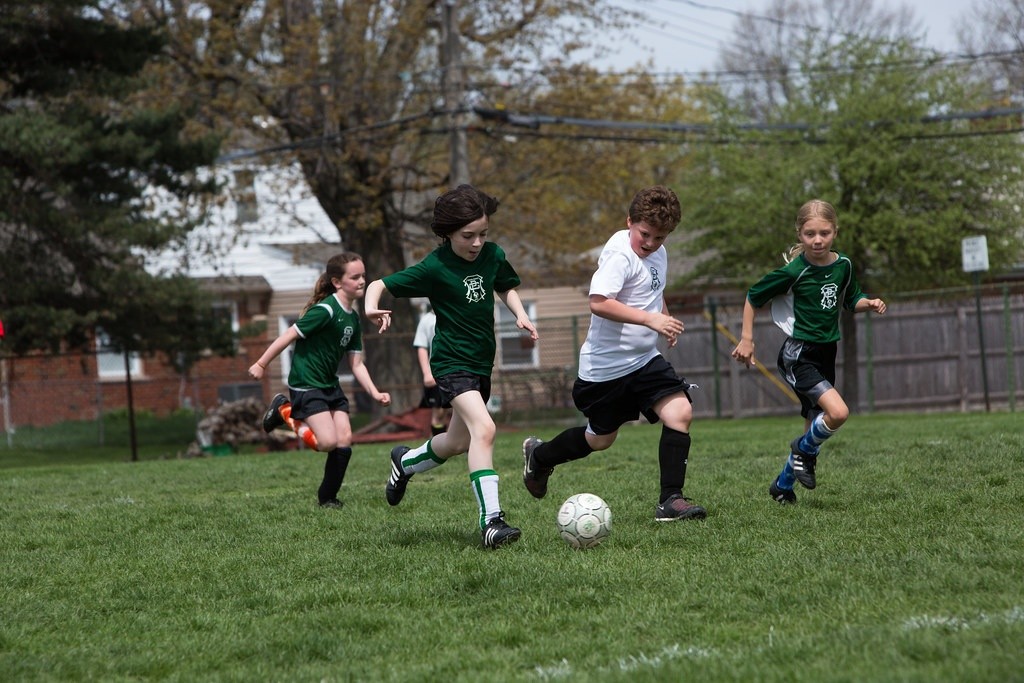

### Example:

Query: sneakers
xmin=261 ymin=392 xmax=289 ymax=433
xmin=653 ymin=494 xmax=707 ymax=521
xmin=768 ymin=474 xmax=797 ymax=506
xmin=790 ymin=434 xmax=820 ymax=489
xmin=384 ymin=446 xmax=416 ymax=506
xmin=319 ymin=498 xmax=343 ymax=509
xmin=522 ymin=435 xmax=555 ymax=499
xmin=481 ymin=510 xmax=521 ymax=549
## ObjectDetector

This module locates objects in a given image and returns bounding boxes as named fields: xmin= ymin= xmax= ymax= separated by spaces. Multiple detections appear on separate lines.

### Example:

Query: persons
xmin=365 ymin=184 xmax=523 ymax=549
xmin=412 ymin=308 xmax=453 ymax=435
xmin=522 ymin=187 xmax=706 ymax=520
xmin=733 ymin=200 xmax=887 ymax=502
xmin=248 ymin=252 xmax=391 ymax=503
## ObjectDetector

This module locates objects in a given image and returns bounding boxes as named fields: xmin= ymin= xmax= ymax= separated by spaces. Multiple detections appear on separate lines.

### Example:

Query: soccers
xmin=554 ymin=492 xmax=614 ymax=550
